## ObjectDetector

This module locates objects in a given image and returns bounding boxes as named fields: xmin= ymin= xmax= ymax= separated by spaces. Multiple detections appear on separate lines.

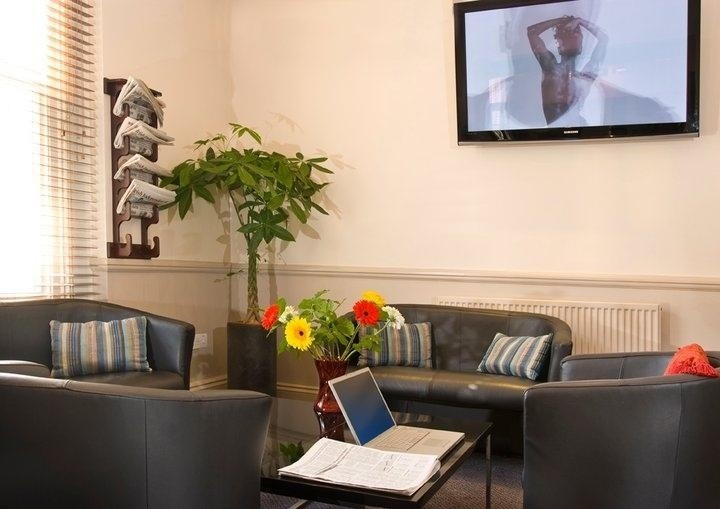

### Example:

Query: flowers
xmin=253 ymin=283 xmax=408 ymax=368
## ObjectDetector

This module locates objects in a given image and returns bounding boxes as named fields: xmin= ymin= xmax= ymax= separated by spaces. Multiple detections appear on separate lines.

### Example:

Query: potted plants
xmin=149 ymin=116 xmax=339 ymax=402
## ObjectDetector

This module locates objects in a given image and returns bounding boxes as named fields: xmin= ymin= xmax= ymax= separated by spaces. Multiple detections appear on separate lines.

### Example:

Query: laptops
xmin=328 ymin=366 xmax=465 ymax=460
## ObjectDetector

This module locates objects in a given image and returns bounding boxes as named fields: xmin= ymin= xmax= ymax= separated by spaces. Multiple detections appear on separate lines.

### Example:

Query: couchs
xmin=307 ymin=300 xmax=575 ymax=464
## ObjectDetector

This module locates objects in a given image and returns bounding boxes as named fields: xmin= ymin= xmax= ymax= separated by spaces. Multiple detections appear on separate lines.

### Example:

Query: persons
xmin=468 ymin=0 xmax=680 ymax=131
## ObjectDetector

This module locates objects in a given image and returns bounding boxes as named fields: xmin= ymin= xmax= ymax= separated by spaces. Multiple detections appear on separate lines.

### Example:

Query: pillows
xmin=46 ymin=313 xmax=155 ymax=381
xmin=660 ymin=341 xmax=720 ymax=382
xmin=351 ymin=314 xmax=436 ymax=372
xmin=473 ymin=327 xmax=555 ymax=384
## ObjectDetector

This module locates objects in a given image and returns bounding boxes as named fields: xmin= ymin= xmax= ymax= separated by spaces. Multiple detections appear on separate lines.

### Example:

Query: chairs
xmin=0 ymin=357 xmax=277 ymax=509
xmin=0 ymin=293 xmax=197 ymax=394
xmin=518 ymin=347 xmax=719 ymax=509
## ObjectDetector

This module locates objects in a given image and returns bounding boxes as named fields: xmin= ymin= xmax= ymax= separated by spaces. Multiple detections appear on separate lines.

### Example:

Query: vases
xmin=309 ymin=350 xmax=355 ymax=416
xmin=311 ymin=402 xmax=348 ymax=443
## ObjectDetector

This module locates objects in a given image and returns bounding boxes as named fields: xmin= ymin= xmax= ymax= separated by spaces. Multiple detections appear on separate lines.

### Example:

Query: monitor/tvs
xmin=454 ymin=0 xmax=701 ymax=145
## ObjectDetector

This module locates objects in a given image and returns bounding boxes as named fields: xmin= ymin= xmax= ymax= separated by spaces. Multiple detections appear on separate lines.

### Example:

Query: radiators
xmin=419 ymin=293 xmax=668 ymax=361
